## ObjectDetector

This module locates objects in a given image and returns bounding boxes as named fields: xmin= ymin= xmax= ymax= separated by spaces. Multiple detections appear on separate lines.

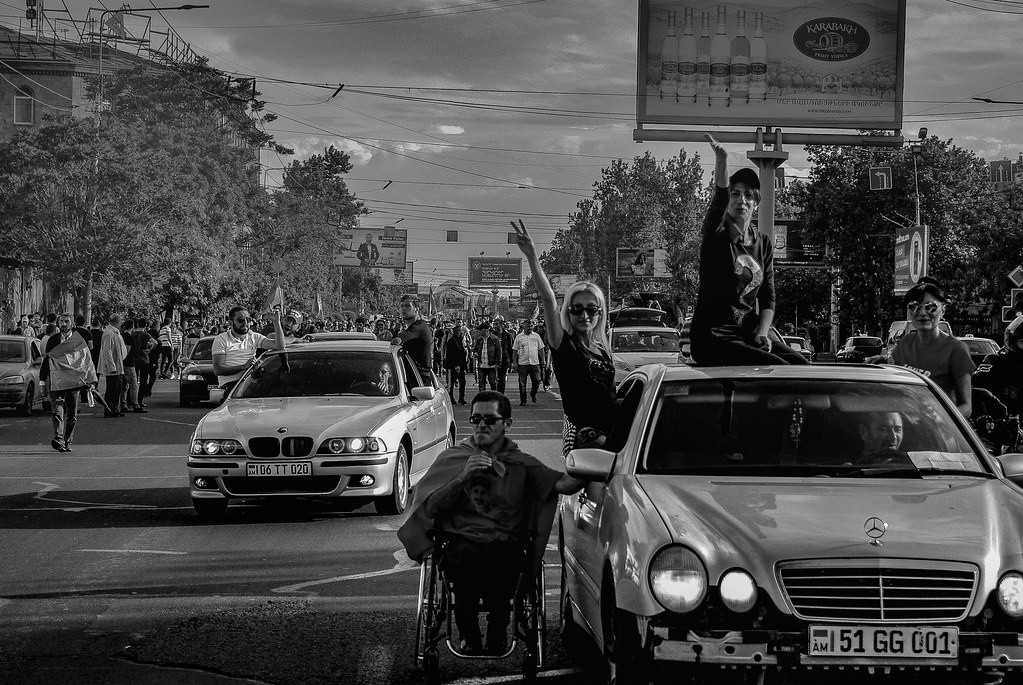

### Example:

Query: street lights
xmin=910 ymin=127 xmax=927 ymax=225
xmin=83 ymin=5 xmax=210 ymax=327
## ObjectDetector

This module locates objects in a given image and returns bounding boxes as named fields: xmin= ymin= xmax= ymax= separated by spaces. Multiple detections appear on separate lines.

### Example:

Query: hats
xmin=730 ymin=167 xmax=760 ymax=190
xmin=475 ymin=323 xmax=490 ymax=331
xmin=285 ymin=309 xmax=303 ymax=324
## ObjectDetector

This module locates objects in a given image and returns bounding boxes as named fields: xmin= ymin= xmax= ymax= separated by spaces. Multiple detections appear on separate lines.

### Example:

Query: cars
xmin=954 ymin=336 xmax=1004 ymax=367
xmin=834 ymin=337 xmax=884 ymax=364
xmin=179 ymin=336 xmax=219 ymax=404
xmin=558 ymin=362 xmax=1023 ymax=685
xmin=606 ymin=307 xmax=682 ymax=391
xmin=186 ymin=340 xmax=457 ymax=515
xmin=0 ymin=335 xmax=51 ymax=415
xmin=768 ymin=325 xmax=811 ymax=360
xmin=302 ymin=332 xmax=377 ymax=341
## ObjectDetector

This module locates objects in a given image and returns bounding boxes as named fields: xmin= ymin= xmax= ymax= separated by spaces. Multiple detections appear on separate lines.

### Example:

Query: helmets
xmin=1004 ymin=315 xmax=1023 ymax=356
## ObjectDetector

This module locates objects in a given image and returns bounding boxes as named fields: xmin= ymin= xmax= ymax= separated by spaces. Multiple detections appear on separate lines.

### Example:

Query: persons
xmin=512 ymin=318 xmax=553 ymax=407
xmin=797 ymin=322 xmax=819 ymax=360
xmin=391 ymin=294 xmax=434 ymax=388
xmin=634 ymin=253 xmax=646 ymax=265
xmin=510 ymin=219 xmax=617 ymax=457
xmin=426 ymin=316 xmax=517 ymax=404
xmin=835 ymin=410 xmax=903 ymax=468
xmin=396 ymin=390 xmax=590 ymax=656
xmin=971 ymin=314 xmax=1023 ymax=428
xmin=689 ymin=134 xmax=810 ymax=460
xmin=357 ymin=233 xmax=380 ymax=264
xmin=261 ymin=309 xmax=406 ymax=340
xmin=550 ymin=274 xmax=560 ymax=291
xmin=15 ymin=308 xmax=174 ymax=453
xmin=362 ymin=359 xmax=393 ymax=396
xmin=889 ymin=277 xmax=976 ymax=418
xmin=154 ymin=303 xmax=286 ymax=403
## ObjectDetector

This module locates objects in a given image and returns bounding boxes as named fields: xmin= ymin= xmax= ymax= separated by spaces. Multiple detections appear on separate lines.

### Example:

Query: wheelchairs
xmin=413 ymin=545 xmax=547 ymax=682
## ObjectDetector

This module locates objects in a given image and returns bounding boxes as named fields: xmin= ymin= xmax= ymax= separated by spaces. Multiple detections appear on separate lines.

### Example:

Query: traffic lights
xmin=1001 ymin=289 xmax=1023 ymax=322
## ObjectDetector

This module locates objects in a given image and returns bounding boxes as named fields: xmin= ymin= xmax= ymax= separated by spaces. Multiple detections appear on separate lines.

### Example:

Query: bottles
xmin=707 ymin=5 xmax=731 ymax=106
xmin=87 ymin=388 xmax=95 ymax=407
xmin=678 ymin=7 xmax=697 ymax=103
xmin=660 ymin=10 xmax=679 ymax=101
xmin=748 ymin=12 xmax=767 ymax=100
xmin=730 ymin=10 xmax=751 ymax=103
xmin=696 ymin=11 xmax=712 ymax=104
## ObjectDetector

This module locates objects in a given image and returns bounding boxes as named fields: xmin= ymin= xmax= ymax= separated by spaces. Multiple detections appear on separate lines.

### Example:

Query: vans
xmin=881 ymin=320 xmax=953 ymax=360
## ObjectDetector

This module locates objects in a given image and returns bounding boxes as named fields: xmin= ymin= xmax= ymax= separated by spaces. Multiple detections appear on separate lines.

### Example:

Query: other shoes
xmin=63 ymin=443 xmax=72 ymax=451
xmin=461 ymin=631 xmax=482 ymax=654
xmin=435 ymin=373 xmax=549 ymax=405
xmin=485 ymin=620 xmax=508 ymax=656
xmin=50 ymin=440 xmax=66 ymax=453
xmin=103 ymin=373 xmax=180 ymax=417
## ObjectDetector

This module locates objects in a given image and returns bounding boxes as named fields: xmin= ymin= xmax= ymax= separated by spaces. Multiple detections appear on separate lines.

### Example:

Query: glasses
xmin=908 ymin=300 xmax=943 ymax=313
xmin=236 ymin=316 xmax=252 ymax=323
xmin=377 ymin=324 xmax=383 ymax=326
xmin=469 ymin=415 xmax=505 ymax=425
xmin=568 ymin=305 xmax=602 ymax=316
